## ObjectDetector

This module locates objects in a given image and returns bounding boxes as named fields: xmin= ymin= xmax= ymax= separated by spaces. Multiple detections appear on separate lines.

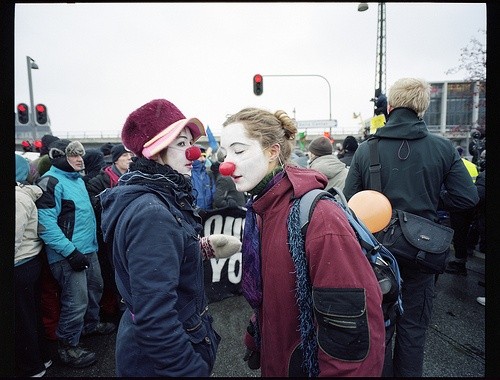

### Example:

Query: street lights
xmin=356 ymin=2 xmax=387 ymax=98
xmin=26 ymin=56 xmax=40 ymax=151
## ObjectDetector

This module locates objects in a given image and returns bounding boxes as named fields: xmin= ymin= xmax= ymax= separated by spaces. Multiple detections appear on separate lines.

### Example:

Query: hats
xmin=120 ymin=97 xmax=206 ymax=159
xmin=338 ymin=136 xmax=358 ymax=152
xmin=48 ymin=139 xmax=86 ymax=172
xmin=112 ymin=145 xmax=131 ymax=162
xmin=40 ymin=135 xmax=60 ymax=156
xmin=100 ymin=142 xmax=114 ymax=155
xmin=309 ymin=136 xmax=332 ymax=155
xmin=16 ymin=154 xmax=32 ymax=182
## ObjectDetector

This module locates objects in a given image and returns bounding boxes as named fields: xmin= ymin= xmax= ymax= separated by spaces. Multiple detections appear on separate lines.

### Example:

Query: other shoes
xmin=477 ymin=297 xmax=485 ymax=306
xmin=445 ymin=261 xmax=468 ymax=276
xmin=16 ymin=356 xmax=51 ymax=378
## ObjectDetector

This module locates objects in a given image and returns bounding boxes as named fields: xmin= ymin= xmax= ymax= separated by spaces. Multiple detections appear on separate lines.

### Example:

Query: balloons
xmin=347 ymin=190 xmax=392 ymax=234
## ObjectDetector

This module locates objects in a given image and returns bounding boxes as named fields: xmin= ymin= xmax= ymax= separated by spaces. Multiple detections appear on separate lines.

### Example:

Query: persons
xmin=342 ymin=77 xmax=481 ymax=377
xmin=218 ymin=108 xmax=386 ymax=377
xmin=15 ymin=127 xmax=485 ymax=378
xmin=99 ymin=98 xmax=242 ymax=377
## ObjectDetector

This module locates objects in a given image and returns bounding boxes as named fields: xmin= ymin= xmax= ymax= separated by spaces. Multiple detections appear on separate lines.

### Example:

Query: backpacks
xmin=298 ymin=187 xmax=404 ymax=354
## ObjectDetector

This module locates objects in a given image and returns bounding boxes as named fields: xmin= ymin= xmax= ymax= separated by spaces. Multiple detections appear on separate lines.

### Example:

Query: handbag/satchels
xmin=376 ymin=210 xmax=457 ymax=279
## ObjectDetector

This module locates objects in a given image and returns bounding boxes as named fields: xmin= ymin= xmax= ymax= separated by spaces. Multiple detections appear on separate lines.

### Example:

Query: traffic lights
xmin=23 ymin=141 xmax=33 ymax=152
xmin=36 ymin=103 xmax=48 ymax=126
xmin=252 ymin=74 xmax=264 ymax=95
xmin=17 ymin=103 xmax=30 ymax=125
xmin=34 ymin=139 xmax=45 ymax=152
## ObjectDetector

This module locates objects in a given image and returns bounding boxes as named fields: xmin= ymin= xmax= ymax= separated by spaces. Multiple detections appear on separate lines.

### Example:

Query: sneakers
xmin=82 ymin=320 xmax=114 ymax=339
xmin=59 ymin=346 xmax=100 ymax=367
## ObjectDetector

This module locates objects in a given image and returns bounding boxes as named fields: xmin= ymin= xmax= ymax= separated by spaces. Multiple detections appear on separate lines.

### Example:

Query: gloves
xmin=199 ymin=233 xmax=241 ymax=261
xmin=65 ymin=248 xmax=90 ymax=271
